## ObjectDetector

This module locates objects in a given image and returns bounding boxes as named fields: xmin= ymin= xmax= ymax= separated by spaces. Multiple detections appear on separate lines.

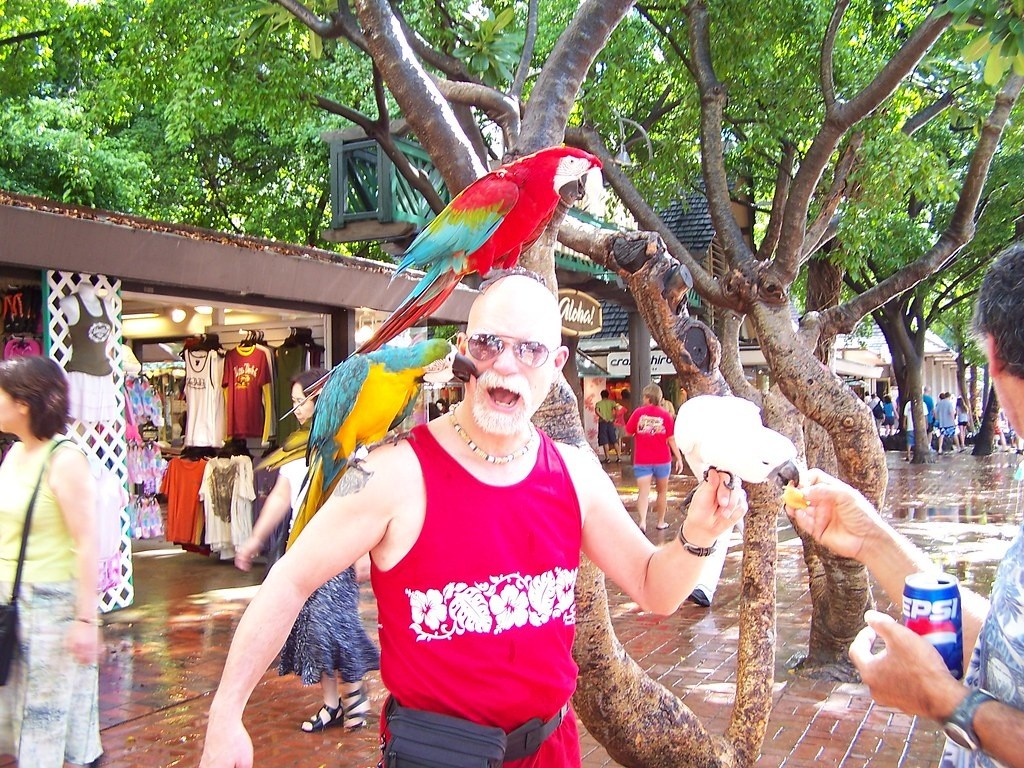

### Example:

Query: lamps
xmin=613 ymin=114 xmax=633 ymax=166
xmin=619 ymin=333 xmax=629 ymax=350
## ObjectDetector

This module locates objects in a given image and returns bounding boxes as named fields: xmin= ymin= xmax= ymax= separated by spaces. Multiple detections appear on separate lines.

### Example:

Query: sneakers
xmin=688 ymin=590 xmax=710 ymax=608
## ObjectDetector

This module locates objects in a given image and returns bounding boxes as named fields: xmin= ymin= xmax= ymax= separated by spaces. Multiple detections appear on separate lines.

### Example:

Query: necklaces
xmin=449 ymin=401 xmax=537 ymax=464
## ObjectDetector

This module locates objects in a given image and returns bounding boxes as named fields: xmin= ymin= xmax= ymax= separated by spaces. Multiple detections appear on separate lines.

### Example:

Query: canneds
xmin=902 ymin=571 xmax=964 ymax=683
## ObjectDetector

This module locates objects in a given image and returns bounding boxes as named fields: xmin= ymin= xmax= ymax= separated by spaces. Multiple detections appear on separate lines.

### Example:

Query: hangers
xmin=127 ymin=437 xmax=166 ymax=448
xmin=142 ymin=415 xmax=159 ymax=431
xmin=261 ymin=435 xmax=279 ymax=457
xmin=137 ymin=493 xmax=160 ymax=503
xmin=179 ymin=333 xmax=228 ymax=361
xmin=218 ymin=439 xmax=254 ymax=460
xmin=239 ymin=329 xmax=268 ymax=347
xmin=132 ymin=373 xmax=152 ymax=384
xmin=181 ymin=446 xmax=216 ymax=460
xmin=281 ymin=328 xmax=325 ymax=352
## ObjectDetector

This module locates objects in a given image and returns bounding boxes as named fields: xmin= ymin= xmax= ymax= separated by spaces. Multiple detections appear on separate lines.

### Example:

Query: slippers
xmin=656 ymin=523 xmax=669 ymax=530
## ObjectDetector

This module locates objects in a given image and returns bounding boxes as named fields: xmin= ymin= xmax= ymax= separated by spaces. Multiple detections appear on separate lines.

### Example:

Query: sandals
xmin=301 ymin=703 xmax=344 ymax=731
xmin=340 ymin=688 xmax=367 ymax=728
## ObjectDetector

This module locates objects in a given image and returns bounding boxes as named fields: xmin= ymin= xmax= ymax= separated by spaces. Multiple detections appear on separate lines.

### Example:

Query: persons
xmin=615 ymin=389 xmax=631 ymax=454
xmin=688 ymin=517 xmax=744 ymax=607
xmin=595 ymin=390 xmax=621 ymax=464
xmin=235 ymin=369 xmax=379 ymax=732
xmin=0 ymin=356 xmax=104 ymax=768
xmin=199 ymin=275 xmax=748 ymax=768
xmin=661 ymin=399 xmax=674 ymax=415
xmin=993 ymin=410 xmax=1020 ymax=451
xmin=785 ymin=242 xmax=1024 ymax=768
xmin=903 ymin=386 xmax=969 ymax=462
xmin=864 ymin=391 xmax=900 ymax=436
xmin=625 ymin=384 xmax=683 ymax=534
xmin=429 ymin=398 xmax=458 ymax=421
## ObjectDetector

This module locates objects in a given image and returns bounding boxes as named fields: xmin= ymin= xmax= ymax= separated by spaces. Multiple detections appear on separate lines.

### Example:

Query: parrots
xmin=673 ymin=393 xmax=800 ymax=490
xmin=280 ymin=147 xmax=603 ymax=430
xmin=285 ymin=340 xmax=479 ymax=558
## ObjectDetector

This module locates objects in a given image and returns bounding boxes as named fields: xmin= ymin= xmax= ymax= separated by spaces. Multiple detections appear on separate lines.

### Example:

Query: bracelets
xmin=79 ymin=617 xmax=102 ymax=625
xmin=679 ymin=523 xmax=720 ymax=558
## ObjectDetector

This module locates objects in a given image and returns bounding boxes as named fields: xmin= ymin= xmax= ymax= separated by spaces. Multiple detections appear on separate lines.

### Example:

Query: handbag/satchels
xmin=384 ymin=695 xmax=567 ymax=768
xmin=0 ymin=604 xmax=18 ymax=686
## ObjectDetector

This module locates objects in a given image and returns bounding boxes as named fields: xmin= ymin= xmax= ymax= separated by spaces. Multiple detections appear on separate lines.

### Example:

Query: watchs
xmin=943 ymin=687 xmax=999 ymax=752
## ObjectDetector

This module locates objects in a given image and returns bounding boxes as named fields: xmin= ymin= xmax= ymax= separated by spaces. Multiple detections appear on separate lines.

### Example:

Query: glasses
xmin=464 ymin=334 xmax=559 ymax=368
